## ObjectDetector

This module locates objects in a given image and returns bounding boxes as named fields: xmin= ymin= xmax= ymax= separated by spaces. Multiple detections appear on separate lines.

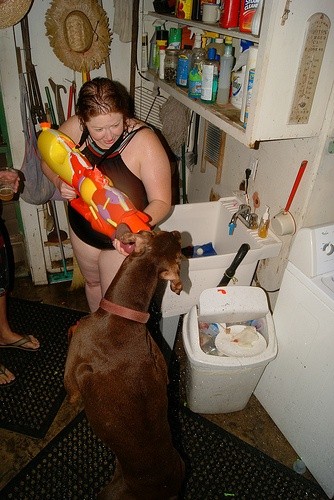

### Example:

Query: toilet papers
xmin=233 ymin=46 xmax=258 ymax=123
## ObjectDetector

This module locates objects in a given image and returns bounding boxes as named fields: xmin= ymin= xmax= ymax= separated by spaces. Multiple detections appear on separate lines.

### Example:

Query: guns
xmin=32 ymin=126 xmax=153 ymax=254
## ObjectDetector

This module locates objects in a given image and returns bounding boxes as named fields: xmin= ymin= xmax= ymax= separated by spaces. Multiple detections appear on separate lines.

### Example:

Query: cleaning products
xmin=258 ymin=204 xmax=271 ymax=239
xmin=139 ymin=0 xmax=263 ymax=119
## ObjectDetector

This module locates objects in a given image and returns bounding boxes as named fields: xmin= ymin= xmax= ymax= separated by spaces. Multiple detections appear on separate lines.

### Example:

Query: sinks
xmin=156 ymin=196 xmax=283 ymax=318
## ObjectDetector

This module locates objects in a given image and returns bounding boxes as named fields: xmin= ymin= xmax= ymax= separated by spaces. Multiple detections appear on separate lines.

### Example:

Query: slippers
xmin=0 ymin=334 xmax=39 ymax=351
xmin=0 ymin=364 xmax=17 ymax=387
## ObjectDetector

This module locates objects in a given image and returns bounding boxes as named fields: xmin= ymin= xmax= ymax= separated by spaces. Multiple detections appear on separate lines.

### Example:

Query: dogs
xmin=62 ymin=230 xmax=186 ymax=500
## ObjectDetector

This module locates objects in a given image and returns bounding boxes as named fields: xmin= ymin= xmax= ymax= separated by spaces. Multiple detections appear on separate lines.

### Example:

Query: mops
xmin=159 ymin=96 xmax=191 ymax=205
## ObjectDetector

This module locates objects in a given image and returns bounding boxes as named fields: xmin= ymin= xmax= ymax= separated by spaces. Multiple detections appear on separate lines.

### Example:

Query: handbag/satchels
xmin=17 ymin=70 xmax=56 ymax=204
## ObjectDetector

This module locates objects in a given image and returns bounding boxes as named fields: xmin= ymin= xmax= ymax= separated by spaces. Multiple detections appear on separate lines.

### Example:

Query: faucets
xmin=228 ymin=203 xmax=259 ymax=236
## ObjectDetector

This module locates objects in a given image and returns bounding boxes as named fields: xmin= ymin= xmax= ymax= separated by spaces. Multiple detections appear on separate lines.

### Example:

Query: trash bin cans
xmin=181 ymin=286 xmax=279 ymax=414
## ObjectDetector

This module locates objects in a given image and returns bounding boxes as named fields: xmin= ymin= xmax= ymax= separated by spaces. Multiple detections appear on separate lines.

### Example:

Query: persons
xmin=0 ymin=170 xmax=41 ymax=388
xmin=40 ymin=78 xmax=171 ymax=313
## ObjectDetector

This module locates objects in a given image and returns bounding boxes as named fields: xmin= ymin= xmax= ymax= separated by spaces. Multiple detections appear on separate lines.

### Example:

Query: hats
xmin=45 ymin=0 xmax=113 ymax=73
xmin=0 ymin=0 xmax=33 ymax=29
